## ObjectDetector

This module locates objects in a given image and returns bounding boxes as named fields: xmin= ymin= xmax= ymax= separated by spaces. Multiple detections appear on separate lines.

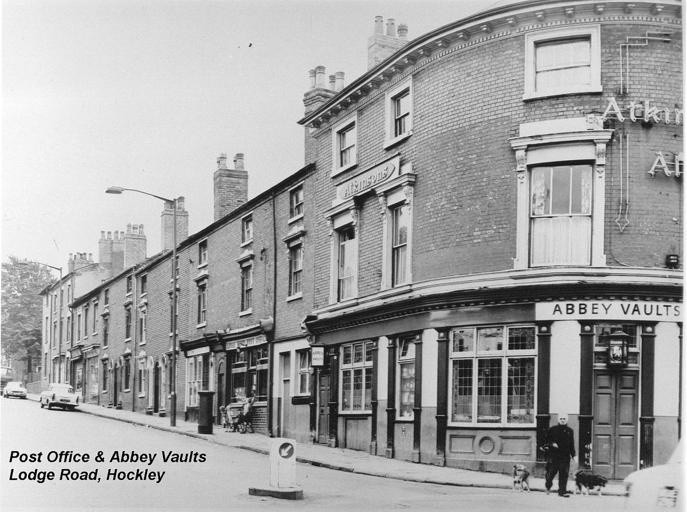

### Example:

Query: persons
xmin=543 ymin=411 xmax=577 ymax=497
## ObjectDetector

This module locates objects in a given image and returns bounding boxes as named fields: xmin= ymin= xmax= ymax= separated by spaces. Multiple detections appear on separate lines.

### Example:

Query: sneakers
xmin=546 ymin=484 xmax=570 ymax=498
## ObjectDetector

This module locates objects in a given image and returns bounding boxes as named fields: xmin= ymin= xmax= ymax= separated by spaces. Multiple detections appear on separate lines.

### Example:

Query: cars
xmin=3 ymin=381 xmax=28 ymax=399
xmin=625 ymin=434 xmax=683 ymax=511
xmin=39 ymin=383 xmax=79 ymax=411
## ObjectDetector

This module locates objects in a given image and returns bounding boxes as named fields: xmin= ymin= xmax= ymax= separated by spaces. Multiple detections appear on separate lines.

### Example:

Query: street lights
xmin=18 ymin=260 xmax=63 ymax=384
xmin=105 ymin=183 xmax=182 ymax=426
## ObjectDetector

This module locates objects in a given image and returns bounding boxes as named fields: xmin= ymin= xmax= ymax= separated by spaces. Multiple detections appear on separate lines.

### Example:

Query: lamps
xmin=663 ymin=254 xmax=679 ymax=270
xmin=608 ymin=328 xmax=629 ymax=370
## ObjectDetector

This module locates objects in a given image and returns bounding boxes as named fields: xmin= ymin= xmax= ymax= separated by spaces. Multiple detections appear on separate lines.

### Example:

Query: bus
xmin=0 ymin=367 xmax=15 ymax=395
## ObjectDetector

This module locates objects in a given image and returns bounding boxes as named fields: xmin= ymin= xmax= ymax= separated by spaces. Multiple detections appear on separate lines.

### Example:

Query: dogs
xmin=573 ymin=469 xmax=607 ymax=496
xmin=512 ymin=464 xmax=530 ymax=495
